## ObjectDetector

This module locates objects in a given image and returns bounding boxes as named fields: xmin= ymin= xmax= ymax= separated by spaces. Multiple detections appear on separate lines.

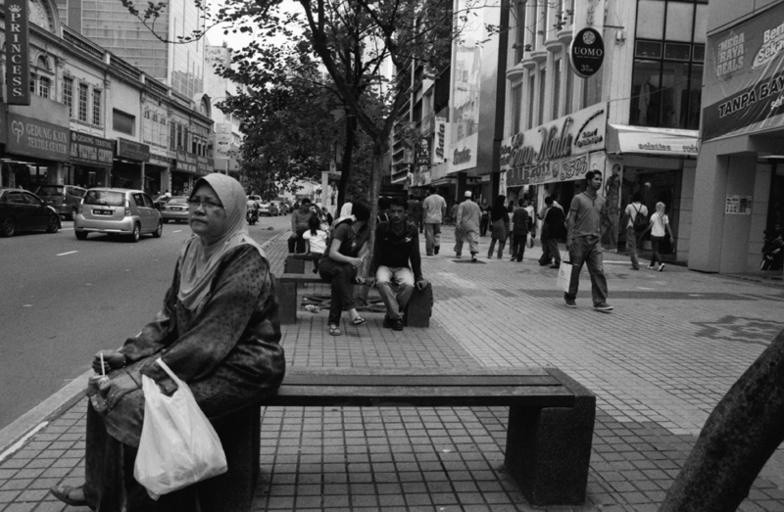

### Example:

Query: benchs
xmin=201 ymin=367 xmax=597 ymax=510
xmin=272 ymin=273 xmax=432 ymax=329
xmin=282 ymin=252 xmax=328 ymax=274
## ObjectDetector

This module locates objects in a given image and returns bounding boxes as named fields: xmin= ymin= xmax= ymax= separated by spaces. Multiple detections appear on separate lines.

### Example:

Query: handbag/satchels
xmin=555 ymin=250 xmax=579 ymax=294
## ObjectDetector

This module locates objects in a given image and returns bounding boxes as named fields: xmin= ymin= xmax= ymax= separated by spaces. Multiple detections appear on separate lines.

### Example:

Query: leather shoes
xmin=390 ymin=318 xmax=403 ymax=331
xmin=383 ymin=316 xmax=391 ymax=328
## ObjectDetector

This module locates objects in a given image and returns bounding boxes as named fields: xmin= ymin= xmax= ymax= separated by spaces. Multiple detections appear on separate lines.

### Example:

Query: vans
xmin=34 ymin=184 xmax=87 ymax=222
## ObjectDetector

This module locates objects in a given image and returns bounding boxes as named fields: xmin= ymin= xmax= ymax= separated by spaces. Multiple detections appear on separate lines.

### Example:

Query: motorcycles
xmin=246 ymin=204 xmax=260 ymax=225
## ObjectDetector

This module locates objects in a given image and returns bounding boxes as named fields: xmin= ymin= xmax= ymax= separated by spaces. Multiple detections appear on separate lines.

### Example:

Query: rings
xmin=112 ymin=394 xmax=115 ymax=398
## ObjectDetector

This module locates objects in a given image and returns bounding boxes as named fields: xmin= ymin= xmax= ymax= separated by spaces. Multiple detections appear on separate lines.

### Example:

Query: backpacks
xmin=629 ymin=203 xmax=649 ymax=232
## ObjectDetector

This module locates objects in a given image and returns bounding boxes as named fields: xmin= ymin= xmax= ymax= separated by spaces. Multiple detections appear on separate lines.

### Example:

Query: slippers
xmin=352 ymin=316 xmax=366 ymax=325
xmin=328 ymin=325 xmax=340 ymax=335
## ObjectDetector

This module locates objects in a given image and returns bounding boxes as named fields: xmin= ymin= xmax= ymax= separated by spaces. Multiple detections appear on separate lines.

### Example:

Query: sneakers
xmin=564 ymin=297 xmax=576 ymax=307
xmin=593 ymin=301 xmax=613 ymax=312
xmin=657 ymin=262 xmax=664 ymax=272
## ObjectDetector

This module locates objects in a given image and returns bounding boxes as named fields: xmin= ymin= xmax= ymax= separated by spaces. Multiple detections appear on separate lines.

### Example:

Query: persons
xmin=374 ymin=197 xmax=428 ymax=332
xmin=649 ymin=202 xmax=674 ymax=271
xmin=319 ymin=200 xmax=376 ymax=336
xmin=49 ymin=172 xmax=287 ymax=512
xmin=625 ymin=193 xmax=649 ymax=272
xmin=605 ymin=163 xmax=621 ymax=247
xmin=564 ymin=169 xmax=615 ymax=310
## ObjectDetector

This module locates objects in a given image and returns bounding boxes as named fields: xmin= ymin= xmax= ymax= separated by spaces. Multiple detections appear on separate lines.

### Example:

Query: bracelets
xmin=126 ymin=370 xmax=141 ymax=388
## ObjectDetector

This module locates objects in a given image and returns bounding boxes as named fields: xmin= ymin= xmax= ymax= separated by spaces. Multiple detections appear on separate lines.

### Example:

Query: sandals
xmin=50 ymin=484 xmax=86 ymax=506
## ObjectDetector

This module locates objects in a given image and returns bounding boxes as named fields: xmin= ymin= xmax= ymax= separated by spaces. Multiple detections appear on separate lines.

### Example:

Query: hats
xmin=465 ymin=191 xmax=471 ymax=197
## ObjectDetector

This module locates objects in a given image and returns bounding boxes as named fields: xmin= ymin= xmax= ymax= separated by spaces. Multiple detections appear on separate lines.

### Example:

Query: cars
xmin=159 ymin=195 xmax=191 ymax=224
xmin=73 ymin=187 xmax=163 ymax=242
xmin=0 ymin=188 xmax=63 ymax=238
xmin=150 ymin=193 xmax=169 ymax=208
xmin=245 ymin=193 xmax=299 ymax=217
